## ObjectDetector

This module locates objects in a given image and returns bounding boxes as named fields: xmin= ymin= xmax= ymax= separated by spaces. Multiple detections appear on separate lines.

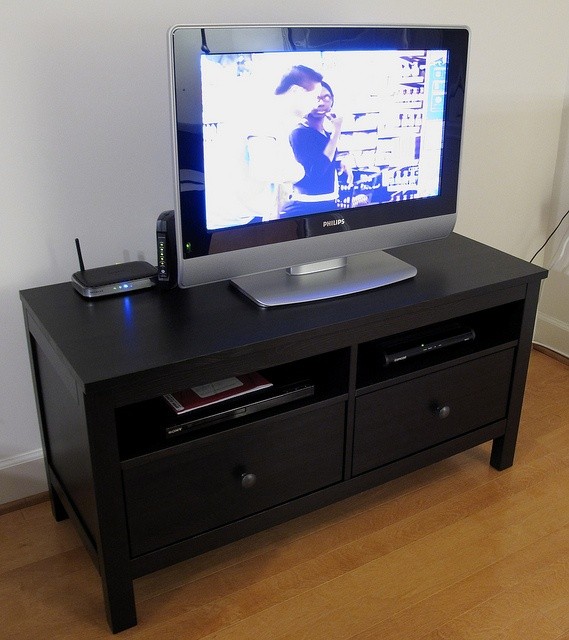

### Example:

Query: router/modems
xmin=70 ymin=238 xmax=159 ymax=297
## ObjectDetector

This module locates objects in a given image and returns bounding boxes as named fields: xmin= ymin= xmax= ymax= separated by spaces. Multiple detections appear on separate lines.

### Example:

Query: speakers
xmin=158 ymin=212 xmax=177 ymax=280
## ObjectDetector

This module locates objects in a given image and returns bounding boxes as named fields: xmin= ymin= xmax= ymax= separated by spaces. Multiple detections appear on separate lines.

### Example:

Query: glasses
xmin=317 ymin=94 xmax=332 ymax=102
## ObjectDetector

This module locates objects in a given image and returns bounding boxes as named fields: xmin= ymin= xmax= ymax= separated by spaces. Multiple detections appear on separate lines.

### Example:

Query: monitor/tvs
xmin=166 ymin=24 xmax=470 ymax=308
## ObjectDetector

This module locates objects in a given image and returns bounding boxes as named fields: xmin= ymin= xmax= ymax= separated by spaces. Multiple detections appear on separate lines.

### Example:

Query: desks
xmin=18 ymin=234 xmax=550 ymax=634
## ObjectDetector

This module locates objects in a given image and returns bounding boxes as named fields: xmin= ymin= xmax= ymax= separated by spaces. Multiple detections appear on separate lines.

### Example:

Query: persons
xmin=245 ymin=65 xmax=322 ymax=224
xmin=284 ymin=83 xmax=344 ymax=219
